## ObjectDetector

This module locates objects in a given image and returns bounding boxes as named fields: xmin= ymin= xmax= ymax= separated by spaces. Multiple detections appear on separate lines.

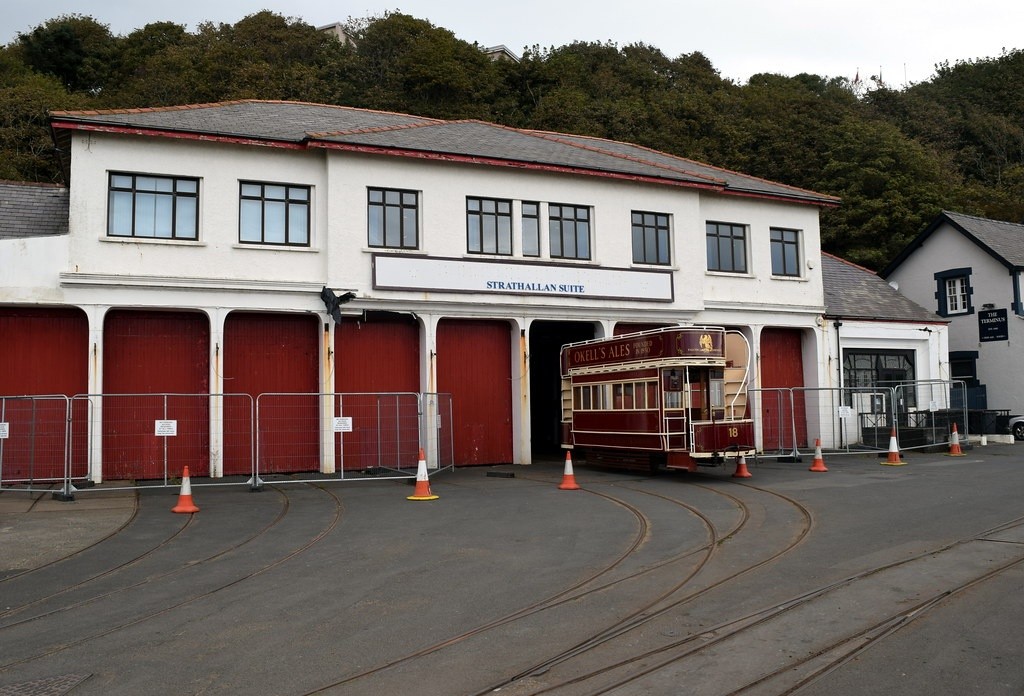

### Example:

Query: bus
xmin=559 ymin=324 xmax=756 ymax=474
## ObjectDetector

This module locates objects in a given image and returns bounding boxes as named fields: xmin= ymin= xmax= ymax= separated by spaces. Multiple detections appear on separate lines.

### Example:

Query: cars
xmin=1008 ymin=415 xmax=1024 ymax=441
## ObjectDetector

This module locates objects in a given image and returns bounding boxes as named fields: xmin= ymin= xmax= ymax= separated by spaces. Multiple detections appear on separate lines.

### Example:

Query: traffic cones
xmin=807 ymin=438 xmax=829 ymax=472
xmin=731 ymin=456 xmax=753 ymax=479
xmin=405 ymin=448 xmax=439 ymax=501
xmin=943 ymin=423 xmax=967 ymax=458
xmin=169 ymin=465 xmax=201 ymax=513
xmin=556 ymin=449 xmax=582 ymax=490
xmin=880 ymin=426 xmax=909 ymax=466
